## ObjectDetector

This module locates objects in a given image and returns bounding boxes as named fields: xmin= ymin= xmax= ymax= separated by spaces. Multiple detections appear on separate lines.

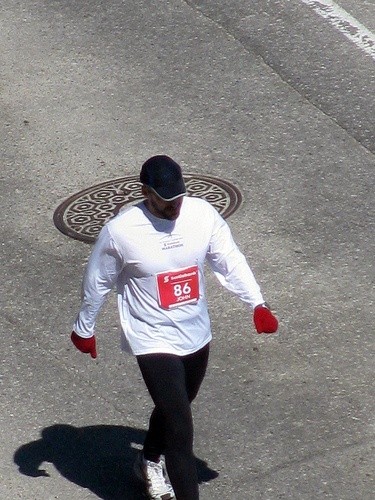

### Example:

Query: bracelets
xmin=254 ymin=302 xmax=271 ymax=311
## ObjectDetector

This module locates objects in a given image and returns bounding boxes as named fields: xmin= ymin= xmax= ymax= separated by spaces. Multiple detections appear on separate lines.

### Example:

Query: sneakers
xmin=133 ymin=449 xmax=177 ymax=500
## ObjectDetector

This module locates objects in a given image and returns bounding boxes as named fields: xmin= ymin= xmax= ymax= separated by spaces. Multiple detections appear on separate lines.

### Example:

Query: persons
xmin=70 ymin=154 xmax=278 ymax=500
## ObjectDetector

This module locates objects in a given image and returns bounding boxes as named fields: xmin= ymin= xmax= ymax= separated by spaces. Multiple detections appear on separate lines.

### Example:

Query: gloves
xmin=71 ymin=331 xmax=97 ymax=359
xmin=254 ymin=307 xmax=279 ymax=334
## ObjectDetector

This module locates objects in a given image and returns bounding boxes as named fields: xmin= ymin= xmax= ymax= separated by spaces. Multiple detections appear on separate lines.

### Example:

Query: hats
xmin=139 ymin=155 xmax=187 ymax=202
